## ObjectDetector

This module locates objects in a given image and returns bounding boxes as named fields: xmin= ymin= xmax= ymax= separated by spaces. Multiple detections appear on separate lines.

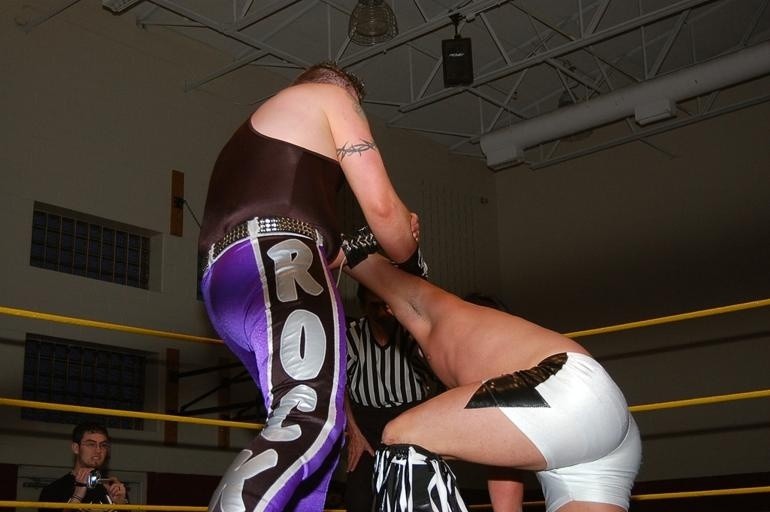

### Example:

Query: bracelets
xmin=73 ymin=494 xmax=83 ymax=501
xmin=74 ymin=480 xmax=88 ymax=487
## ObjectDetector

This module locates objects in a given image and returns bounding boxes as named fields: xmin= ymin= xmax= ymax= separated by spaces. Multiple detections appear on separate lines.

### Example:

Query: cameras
xmin=87 ymin=469 xmax=111 ymax=489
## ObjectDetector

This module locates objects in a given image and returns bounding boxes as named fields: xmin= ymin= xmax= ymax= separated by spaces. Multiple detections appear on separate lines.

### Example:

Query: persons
xmin=39 ymin=420 xmax=132 ymax=512
xmin=329 ymin=246 xmax=643 ymax=511
xmin=191 ymin=64 xmax=421 ymax=511
xmin=328 ymin=282 xmax=433 ymax=511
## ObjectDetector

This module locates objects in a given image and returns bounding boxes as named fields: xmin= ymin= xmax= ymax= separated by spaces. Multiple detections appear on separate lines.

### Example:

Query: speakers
xmin=441 ymin=38 xmax=474 ymax=88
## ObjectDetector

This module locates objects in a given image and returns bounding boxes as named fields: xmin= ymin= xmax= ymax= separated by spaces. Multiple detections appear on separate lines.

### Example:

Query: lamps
xmin=441 ymin=12 xmax=473 ymax=88
xmin=347 ymin=0 xmax=399 ymax=46
